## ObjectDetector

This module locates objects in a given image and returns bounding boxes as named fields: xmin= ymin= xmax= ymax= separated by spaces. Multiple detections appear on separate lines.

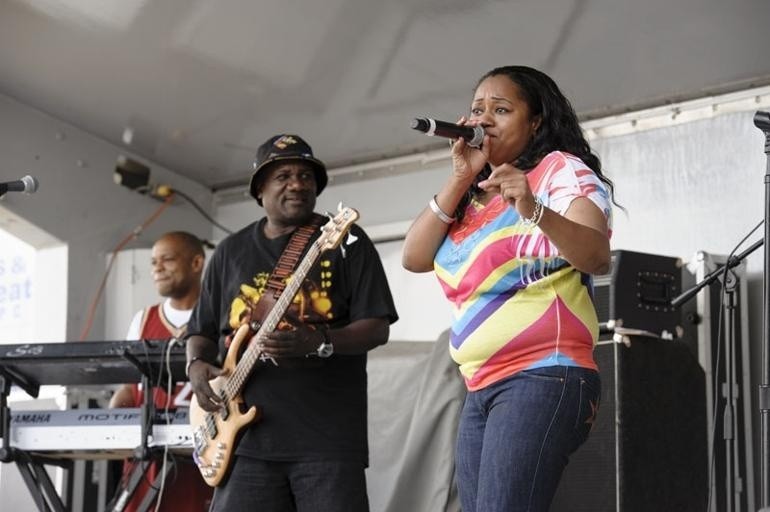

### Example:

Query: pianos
xmin=0 ymin=337 xmax=196 ymax=457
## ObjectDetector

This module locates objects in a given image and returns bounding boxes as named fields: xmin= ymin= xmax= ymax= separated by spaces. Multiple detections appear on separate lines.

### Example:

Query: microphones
xmin=0 ymin=173 xmax=39 ymax=196
xmin=413 ymin=115 xmax=485 ymax=148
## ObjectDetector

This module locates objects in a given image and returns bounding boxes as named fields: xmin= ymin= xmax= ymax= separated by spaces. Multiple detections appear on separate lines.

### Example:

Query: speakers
xmin=549 ymin=334 xmax=704 ymax=511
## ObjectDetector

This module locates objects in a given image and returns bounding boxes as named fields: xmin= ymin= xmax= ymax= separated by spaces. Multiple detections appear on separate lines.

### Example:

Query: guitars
xmin=188 ymin=202 xmax=360 ymax=488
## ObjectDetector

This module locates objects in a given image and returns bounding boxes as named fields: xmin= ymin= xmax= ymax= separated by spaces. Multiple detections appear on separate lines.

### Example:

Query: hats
xmin=246 ymin=132 xmax=329 ymax=207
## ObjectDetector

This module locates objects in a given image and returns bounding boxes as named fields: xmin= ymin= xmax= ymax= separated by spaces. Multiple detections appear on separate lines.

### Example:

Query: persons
xmin=399 ymin=61 xmax=617 ymax=512
xmin=187 ymin=131 xmax=399 ymax=510
xmin=107 ymin=228 xmax=214 ymax=511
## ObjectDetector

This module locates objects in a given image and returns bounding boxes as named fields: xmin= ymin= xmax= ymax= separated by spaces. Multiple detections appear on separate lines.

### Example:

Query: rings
xmin=449 ymin=138 xmax=454 ymax=145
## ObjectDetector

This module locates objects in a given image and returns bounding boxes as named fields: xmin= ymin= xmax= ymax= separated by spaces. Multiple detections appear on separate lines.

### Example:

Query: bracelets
xmin=429 ymin=194 xmax=460 ymax=225
xmin=185 ymin=355 xmax=204 ymax=381
xmin=521 ymin=192 xmax=547 ymax=229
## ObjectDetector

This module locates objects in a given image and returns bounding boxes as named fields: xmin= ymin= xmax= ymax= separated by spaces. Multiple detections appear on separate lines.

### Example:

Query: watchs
xmin=315 ymin=325 xmax=337 ymax=360
